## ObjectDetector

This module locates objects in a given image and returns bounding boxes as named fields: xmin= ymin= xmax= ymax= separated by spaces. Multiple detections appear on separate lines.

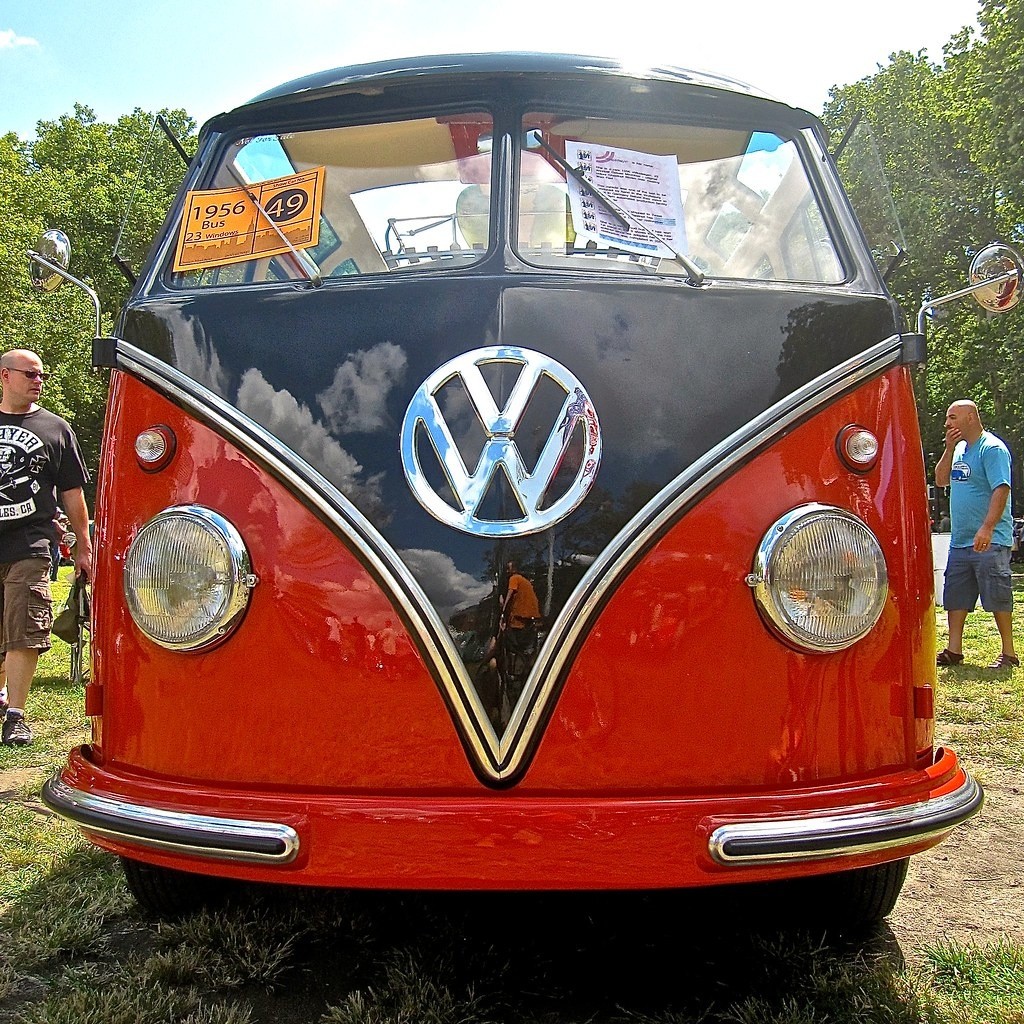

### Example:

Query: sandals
xmin=936 ymin=649 xmax=964 ymax=666
xmin=987 ymin=654 xmax=1020 ymax=669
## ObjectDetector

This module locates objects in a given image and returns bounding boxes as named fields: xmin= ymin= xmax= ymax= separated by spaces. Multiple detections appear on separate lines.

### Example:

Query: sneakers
xmin=0 ymin=692 xmax=9 ymax=719
xmin=2 ymin=711 xmax=32 ymax=746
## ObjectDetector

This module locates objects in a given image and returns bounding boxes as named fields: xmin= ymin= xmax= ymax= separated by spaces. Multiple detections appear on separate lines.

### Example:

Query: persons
xmin=486 ymin=560 xmax=539 ymax=683
xmin=1011 ymin=515 xmax=1024 ymax=564
xmin=50 ymin=506 xmax=94 ymax=582
xmin=936 ymin=511 xmax=951 ymax=532
xmin=0 ymin=349 xmax=93 ymax=747
xmin=935 ymin=399 xmax=1019 ymax=669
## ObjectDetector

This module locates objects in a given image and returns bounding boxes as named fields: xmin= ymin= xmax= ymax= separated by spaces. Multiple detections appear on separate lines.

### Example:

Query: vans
xmin=26 ymin=50 xmax=1024 ymax=933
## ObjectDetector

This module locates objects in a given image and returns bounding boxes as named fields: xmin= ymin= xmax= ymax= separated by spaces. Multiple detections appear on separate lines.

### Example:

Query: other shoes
xmin=1011 ymin=561 xmax=1015 ymax=564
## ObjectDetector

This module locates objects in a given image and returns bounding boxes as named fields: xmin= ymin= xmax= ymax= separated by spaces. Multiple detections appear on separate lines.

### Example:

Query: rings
xmin=983 ymin=544 xmax=986 ymax=546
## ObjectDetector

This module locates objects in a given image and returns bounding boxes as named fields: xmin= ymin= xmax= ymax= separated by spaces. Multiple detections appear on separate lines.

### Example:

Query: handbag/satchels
xmin=1011 ymin=544 xmax=1019 ymax=551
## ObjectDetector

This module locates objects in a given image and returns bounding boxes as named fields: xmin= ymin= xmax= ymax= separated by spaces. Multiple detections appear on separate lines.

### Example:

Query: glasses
xmin=7 ymin=367 xmax=51 ymax=380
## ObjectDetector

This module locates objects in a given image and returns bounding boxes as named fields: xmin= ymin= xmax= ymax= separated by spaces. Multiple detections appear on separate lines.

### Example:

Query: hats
xmin=56 ymin=506 xmax=64 ymax=513
xmin=940 ymin=511 xmax=948 ymax=515
